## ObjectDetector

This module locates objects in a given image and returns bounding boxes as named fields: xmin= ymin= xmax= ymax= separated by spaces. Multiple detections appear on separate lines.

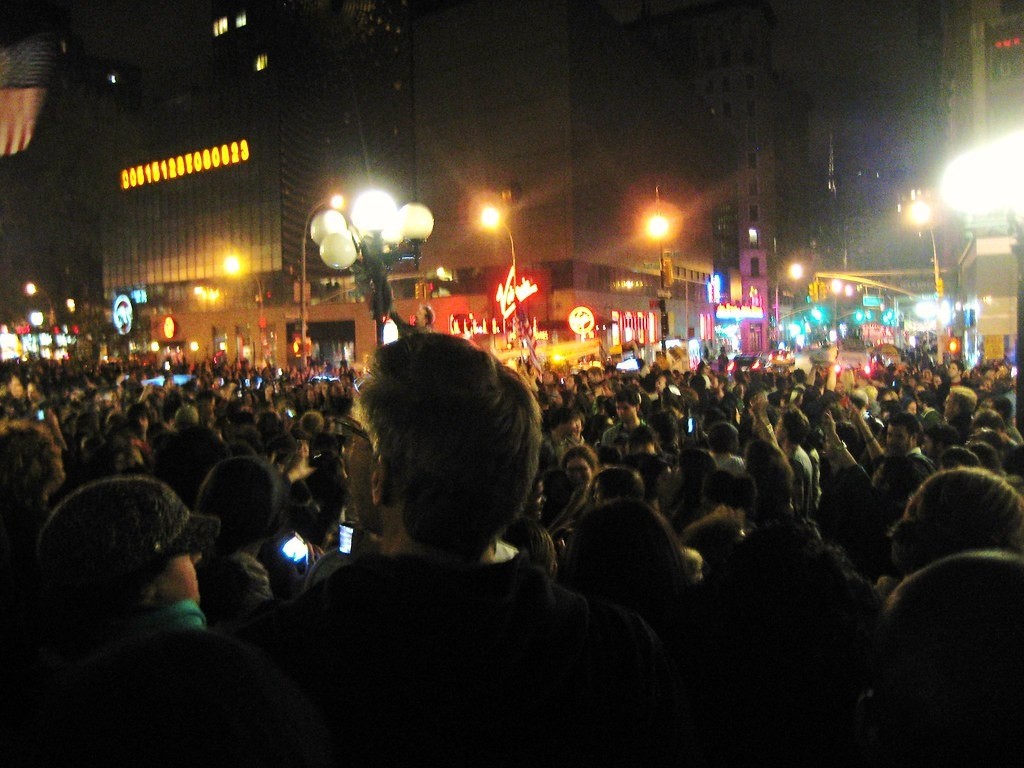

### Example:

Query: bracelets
xmin=834 ymin=440 xmax=847 ymax=451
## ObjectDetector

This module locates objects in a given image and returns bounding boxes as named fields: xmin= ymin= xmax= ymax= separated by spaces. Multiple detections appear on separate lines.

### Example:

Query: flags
xmin=514 ymin=294 xmax=543 ymax=383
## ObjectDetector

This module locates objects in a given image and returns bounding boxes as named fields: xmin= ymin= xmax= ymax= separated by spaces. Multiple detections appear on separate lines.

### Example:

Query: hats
xmin=30 ymin=475 xmax=221 ymax=592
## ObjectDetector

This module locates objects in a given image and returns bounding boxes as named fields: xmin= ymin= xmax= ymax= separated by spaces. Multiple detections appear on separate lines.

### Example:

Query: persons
xmin=1 ymin=332 xmax=1024 ymax=768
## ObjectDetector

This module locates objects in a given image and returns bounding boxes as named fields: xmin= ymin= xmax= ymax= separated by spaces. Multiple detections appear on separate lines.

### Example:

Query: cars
xmin=767 ymin=349 xmax=795 ymax=366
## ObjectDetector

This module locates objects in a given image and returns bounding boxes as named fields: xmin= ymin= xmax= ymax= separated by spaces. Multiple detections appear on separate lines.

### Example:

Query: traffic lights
xmin=934 ymin=276 xmax=945 ymax=298
xmin=947 ymin=338 xmax=962 ymax=354
xmin=809 ymin=281 xmax=819 ymax=304
xmin=820 ymin=282 xmax=829 ymax=302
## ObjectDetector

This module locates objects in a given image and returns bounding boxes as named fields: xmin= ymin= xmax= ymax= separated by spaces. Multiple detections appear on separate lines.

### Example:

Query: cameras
xmin=36 ymin=409 xmax=46 ymax=422
xmin=280 ymin=533 xmax=307 ymax=564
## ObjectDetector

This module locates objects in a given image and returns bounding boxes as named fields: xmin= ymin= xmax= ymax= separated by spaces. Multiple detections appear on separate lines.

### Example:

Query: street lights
xmin=307 ymin=187 xmax=433 ymax=350
xmin=225 ymin=258 xmax=266 ymax=315
xmin=911 ymin=204 xmax=945 ymax=365
xmin=775 ymin=265 xmax=803 ymax=352
xmin=831 ymin=279 xmax=854 ymax=347
xmin=26 ymin=283 xmax=56 ymax=329
xmin=293 ymin=195 xmax=343 ymax=374
xmin=647 ymin=214 xmax=671 ymax=363
xmin=481 ymin=205 xmax=519 ymax=299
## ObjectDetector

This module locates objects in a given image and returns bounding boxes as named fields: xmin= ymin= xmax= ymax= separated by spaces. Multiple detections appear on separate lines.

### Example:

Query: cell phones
xmin=687 ymin=417 xmax=694 ymax=435
xmin=339 ymin=523 xmax=356 ymax=555
